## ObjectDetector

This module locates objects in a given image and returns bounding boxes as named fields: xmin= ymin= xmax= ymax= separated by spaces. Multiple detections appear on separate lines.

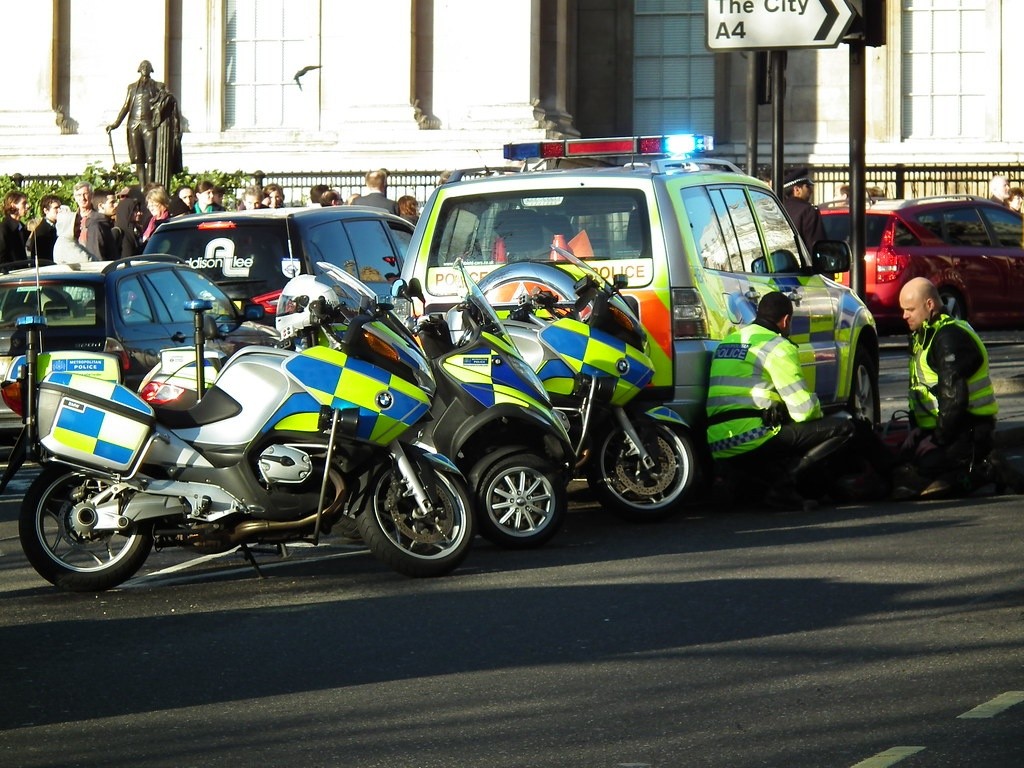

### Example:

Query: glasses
xmin=117 ymin=195 xmax=129 ymax=199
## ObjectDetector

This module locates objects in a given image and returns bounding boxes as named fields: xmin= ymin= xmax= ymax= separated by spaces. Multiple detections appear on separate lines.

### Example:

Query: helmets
xmin=276 ymin=274 xmax=340 ymax=334
xmin=841 ymin=462 xmax=878 ymax=500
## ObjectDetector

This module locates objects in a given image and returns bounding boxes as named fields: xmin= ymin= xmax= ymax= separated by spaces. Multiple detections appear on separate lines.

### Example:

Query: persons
xmin=833 ymin=184 xmax=884 ymax=201
xmin=106 ymin=60 xmax=166 ymax=184
xmin=879 ymin=276 xmax=1008 ymax=501
xmin=755 ymin=167 xmax=835 ymax=281
xmin=705 ymin=292 xmax=856 ymax=513
xmin=989 ymin=175 xmax=1024 ymax=211
xmin=0 ymin=169 xmax=455 ymax=320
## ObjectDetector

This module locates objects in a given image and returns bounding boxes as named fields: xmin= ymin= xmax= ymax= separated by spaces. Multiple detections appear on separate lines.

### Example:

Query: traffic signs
xmin=705 ymin=0 xmax=856 ymax=51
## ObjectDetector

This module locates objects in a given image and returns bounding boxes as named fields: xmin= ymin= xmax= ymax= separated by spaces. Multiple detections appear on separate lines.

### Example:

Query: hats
xmin=169 ymin=196 xmax=188 ymax=217
xmin=783 ymin=168 xmax=814 ymax=189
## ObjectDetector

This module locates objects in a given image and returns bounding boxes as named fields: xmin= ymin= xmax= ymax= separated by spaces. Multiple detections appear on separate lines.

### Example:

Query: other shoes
xmin=987 ymin=450 xmax=1024 ymax=495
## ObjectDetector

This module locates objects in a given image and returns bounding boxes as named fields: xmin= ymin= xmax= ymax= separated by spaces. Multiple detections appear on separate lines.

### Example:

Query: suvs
xmin=394 ymin=134 xmax=883 ymax=434
xmin=814 ymin=194 xmax=1024 ymax=322
xmin=143 ymin=205 xmax=417 ymax=327
xmin=0 ymin=253 xmax=243 ymax=469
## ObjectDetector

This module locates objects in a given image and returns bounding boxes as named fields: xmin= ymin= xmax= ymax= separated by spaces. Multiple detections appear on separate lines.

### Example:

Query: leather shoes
xmin=759 ymin=478 xmax=819 ymax=511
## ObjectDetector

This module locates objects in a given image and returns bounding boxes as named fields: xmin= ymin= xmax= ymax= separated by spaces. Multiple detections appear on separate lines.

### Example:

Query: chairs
xmin=43 ymin=300 xmax=79 ymax=316
xmin=950 ymin=212 xmax=988 ymax=246
xmin=625 ymin=208 xmax=643 ymax=252
xmin=540 ymin=214 xmax=573 ymax=242
xmin=494 ymin=209 xmax=541 ymax=251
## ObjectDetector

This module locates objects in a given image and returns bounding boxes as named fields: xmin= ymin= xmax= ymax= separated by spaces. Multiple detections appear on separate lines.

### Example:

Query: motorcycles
xmin=0 ymin=261 xmax=473 ymax=593
xmin=145 ymin=258 xmax=578 ymax=550
xmin=444 ymin=246 xmax=698 ymax=523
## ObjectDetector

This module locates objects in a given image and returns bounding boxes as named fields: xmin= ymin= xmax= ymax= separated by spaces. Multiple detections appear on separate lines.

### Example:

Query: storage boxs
xmin=157 ymin=346 xmax=227 ymax=389
xmin=39 ymin=372 xmax=156 ymax=474
xmin=6 ymin=350 xmax=123 ymax=386
